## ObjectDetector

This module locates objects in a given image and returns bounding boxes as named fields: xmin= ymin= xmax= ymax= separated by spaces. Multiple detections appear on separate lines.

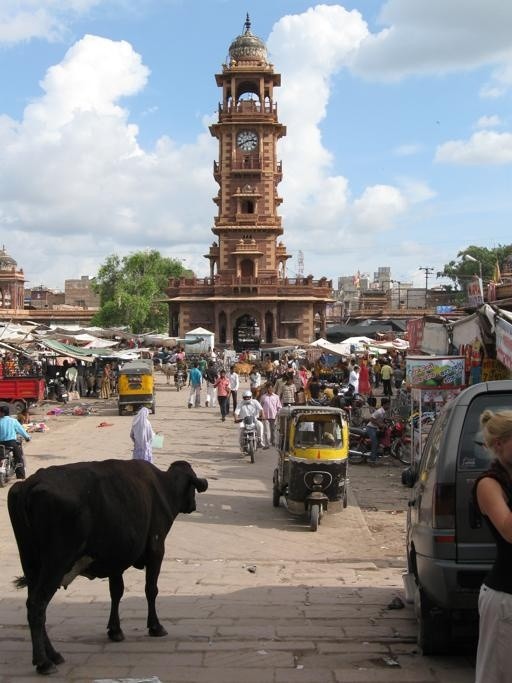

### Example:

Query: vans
xmin=401 ymin=381 xmax=512 ymax=655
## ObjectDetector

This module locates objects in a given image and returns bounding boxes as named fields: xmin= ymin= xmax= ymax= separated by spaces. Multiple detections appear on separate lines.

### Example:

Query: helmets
xmin=242 ymin=390 xmax=253 ymax=398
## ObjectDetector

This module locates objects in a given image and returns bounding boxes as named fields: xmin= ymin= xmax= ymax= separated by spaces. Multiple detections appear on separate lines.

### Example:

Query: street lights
xmin=465 ymin=254 xmax=483 ymax=280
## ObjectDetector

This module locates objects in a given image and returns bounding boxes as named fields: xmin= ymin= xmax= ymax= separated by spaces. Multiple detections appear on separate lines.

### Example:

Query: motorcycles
xmin=234 ymin=415 xmax=262 ymax=463
xmin=348 ymin=419 xmax=412 ymax=465
xmin=0 ymin=436 xmax=23 ymax=486
xmin=49 ymin=371 xmax=70 ymax=404
xmin=173 ymin=366 xmax=188 ymax=392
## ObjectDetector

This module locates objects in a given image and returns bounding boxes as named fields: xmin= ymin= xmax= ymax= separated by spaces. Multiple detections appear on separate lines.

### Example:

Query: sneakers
xmin=258 ymin=442 xmax=265 ymax=449
xmin=240 ymin=446 xmax=245 ymax=453
xmin=196 ymin=404 xmax=202 ymax=407
xmin=187 ymin=403 xmax=192 ymax=408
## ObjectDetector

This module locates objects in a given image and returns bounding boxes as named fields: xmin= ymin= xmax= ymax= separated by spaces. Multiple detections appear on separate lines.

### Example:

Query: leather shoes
xmin=262 ymin=446 xmax=270 ymax=450
xmin=220 ymin=417 xmax=225 ymax=422
xmin=271 ymin=442 xmax=276 ymax=447
xmin=205 ymin=401 xmax=208 ymax=407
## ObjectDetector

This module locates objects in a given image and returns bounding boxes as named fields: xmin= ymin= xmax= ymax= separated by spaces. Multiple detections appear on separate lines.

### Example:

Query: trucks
xmin=1 ymin=377 xmax=45 ymax=413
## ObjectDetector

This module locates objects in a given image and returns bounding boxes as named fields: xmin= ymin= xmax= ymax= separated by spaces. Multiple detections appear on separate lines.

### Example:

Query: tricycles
xmin=273 ymin=404 xmax=348 ymax=531
xmin=118 ymin=359 xmax=155 ymax=415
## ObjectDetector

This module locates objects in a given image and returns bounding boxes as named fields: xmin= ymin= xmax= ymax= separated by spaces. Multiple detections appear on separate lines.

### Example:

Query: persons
xmin=348 ymin=365 xmax=360 ymax=395
xmin=76 ymin=359 xmax=85 ymax=397
xmin=346 ymin=358 xmax=359 ymax=374
xmin=187 ymin=362 xmax=203 ymax=409
xmin=271 ymin=375 xmax=287 ymax=399
xmin=265 ymin=358 xmax=276 ymax=381
xmin=240 ymin=352 xmax=246 ymax=362
xmin=226 ymin=365 xmax=239 ymax=414
xmin=278 ymin=379 xmax=299 ymax=407
xmin=330 ymin=388 xmax=343 ymax=406
xmin=319 ymin=381 xmax=334 ymax=402
xmin=99 ymin=362 xmax=114 ymax=399
xmin=343 ymin=384 xmax=355 ymax=421
xmin=85 ymin=371 xmax=95 ymax=396
xmin=64 ymin=362 xmax=77 ymax=393
xmin=173 ymin=359 xmax=186 ymax=389
xmin=390 ymin=383 xmax=399 ymax=409
xmin=172 ymin=346 xmax=219 ymax=359
xmin=394 ymin=365 xmax=404 ymax=389
xmin=359 ymin=363 xmax=369 ymax=394
xmin=259 ymin=382 xmax=282 ymax=449
xmin=0 ymin=405 xmax=32 ymax=483
xmin=369 ymin=355 xmax=377 ymax=367
xmin=130 ymin=407 xmax=154 ymax=463
xmin=203 ymin=360 xmax=219 ymax=408
xmin=212 ymin=370 xmax=230 ymax=422
xmin=282 ymin=350 xmax=289 ymax=363
xmin=381 ymin=346 xmax=405 ymax=373
xmin=246 ymin=350 xmax=252 ymax=361
xmin=234 ymin=390 xmax=266 ymax=458
xmin=471 ymin=403 xmax=511 ymax=683
xmin=366 ymin=398 xmax=391 ymax=462
xmin=371 ymin=360 xmax=381 ymax=389
xmin=307 ymin=374 xmax=321 ymax=400
xmin=250 ymin=370 xmax=261 ymax=399
xmin=359 ymin=397 xmax=378 ymax=423
xmin=380 ymin=360 xmax=394 ymax=396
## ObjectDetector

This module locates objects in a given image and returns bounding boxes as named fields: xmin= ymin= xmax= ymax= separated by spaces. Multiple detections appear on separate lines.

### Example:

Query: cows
xmin=159 ymin=362 xmax=178 ymax=385
xmin=233 ymin=362 xmax=257 ymax=382
xmin=7 ymin=458 xmax=209 ymax=675
